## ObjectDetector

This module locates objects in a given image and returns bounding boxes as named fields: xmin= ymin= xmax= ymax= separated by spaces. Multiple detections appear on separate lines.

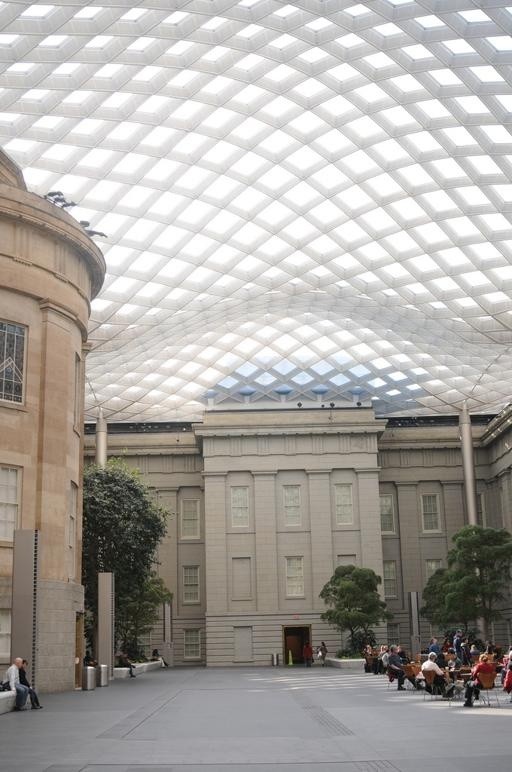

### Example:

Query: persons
xmin=302 ymin=639 xmax=314 ymax=669
xmin=84 ymin=651 xmax=93 ymax=661
xmin=3 ymin=657 xmax=30 ymax=710
xmin=152 ymin=648 xmax=170 ymax=666
xmin=317 ymin=641 xmax=328 ymax=667
xmin=119 ymin=652 xmax=137 ymax=677
xmin=20 ymin=659 xmax=43 ymax=709
xmin=365 ymin=628 xmax=512 ymax=707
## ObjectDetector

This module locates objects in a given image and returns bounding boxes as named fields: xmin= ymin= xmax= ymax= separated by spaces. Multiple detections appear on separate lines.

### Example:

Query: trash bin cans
xmin=272 ymin=653 xmax=278 ymax=666
xmin=97 ymin=665 xmax=109 ymax=687
xmin=82 ymin=666 xmax=97 ymax=690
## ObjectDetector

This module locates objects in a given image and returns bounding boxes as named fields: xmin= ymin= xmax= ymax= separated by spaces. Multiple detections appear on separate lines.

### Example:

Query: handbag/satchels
xmin=318 ymin=651 xmax=322 ymax=658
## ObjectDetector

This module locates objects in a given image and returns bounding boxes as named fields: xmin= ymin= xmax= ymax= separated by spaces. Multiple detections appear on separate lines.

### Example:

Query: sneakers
xmin=464 ymin=701 xmax=473 ymax=707
xmin=416 ymin=685 xmax=425 ymax=689
xmin=16 ymin=705 xmax=43 ymax=711
xmin=475 ymin=696 xmax=480 ymax=700
xmin=398 ymin=685 xmax=406 ymax=690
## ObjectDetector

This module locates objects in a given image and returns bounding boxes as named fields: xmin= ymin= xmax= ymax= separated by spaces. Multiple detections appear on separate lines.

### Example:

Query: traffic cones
xmin=287 ymin=649 xmax=295 ymax=665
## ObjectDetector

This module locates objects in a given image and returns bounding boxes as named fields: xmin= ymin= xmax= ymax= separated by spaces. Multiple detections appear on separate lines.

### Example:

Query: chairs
xmin=385 ymin=652 xmax=512 ymax=708
